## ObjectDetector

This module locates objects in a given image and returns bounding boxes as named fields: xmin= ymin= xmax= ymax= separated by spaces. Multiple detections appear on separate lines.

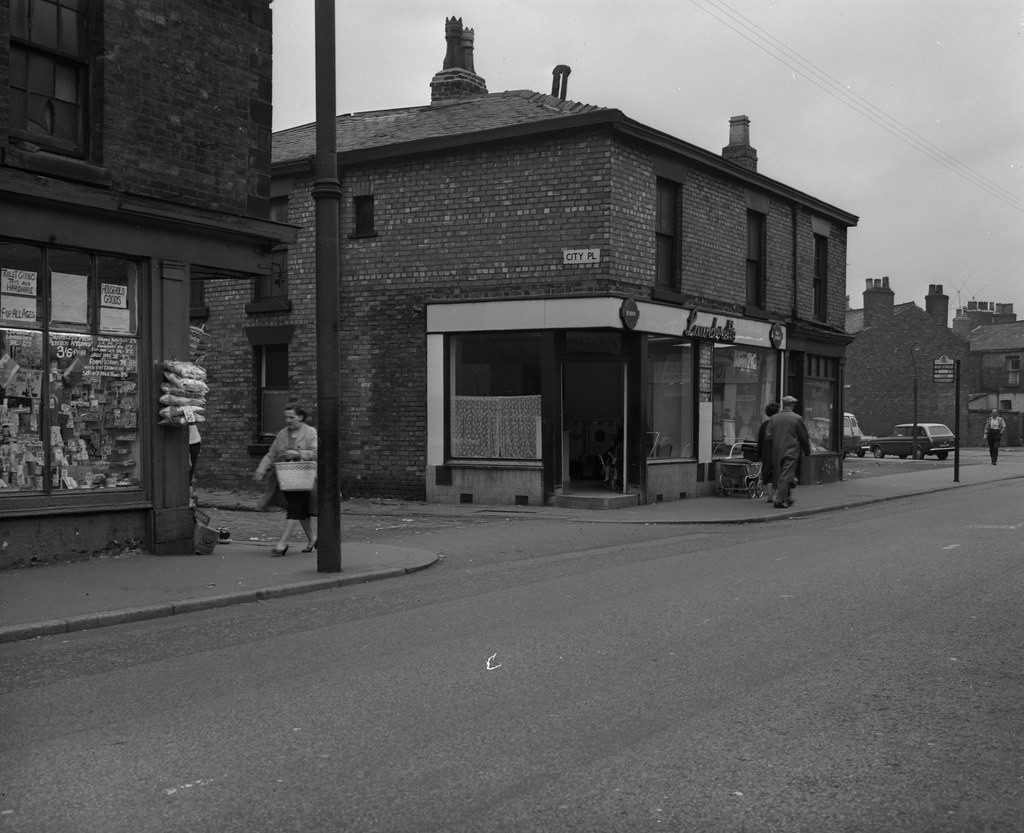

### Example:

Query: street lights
xmin=910 ymin=340 xmax=923 ymax=459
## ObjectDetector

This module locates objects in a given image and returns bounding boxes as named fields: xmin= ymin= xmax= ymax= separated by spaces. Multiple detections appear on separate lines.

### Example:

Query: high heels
xmin=271 ymin=544 xmax=289 ymax=557
xmin=302 ymin=538 xmax=319 ymax=553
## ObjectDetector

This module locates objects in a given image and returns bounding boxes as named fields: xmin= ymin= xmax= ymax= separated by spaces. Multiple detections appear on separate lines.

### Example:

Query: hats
xmin=782 ymin=396 xmax=798 ymax=405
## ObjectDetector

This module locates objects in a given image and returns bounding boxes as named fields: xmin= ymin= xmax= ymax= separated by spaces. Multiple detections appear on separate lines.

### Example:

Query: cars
xmin=843 ymin=412 xmax=877 ymax=458
xmin=870 ymin=423 xmax=955 ymax=462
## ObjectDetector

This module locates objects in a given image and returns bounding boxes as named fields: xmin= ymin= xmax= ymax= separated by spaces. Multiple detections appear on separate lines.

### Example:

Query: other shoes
xmin=787 ymin=501 xmax=794 ymax=506
xmin=774 ymin=501 xmax=788 ymax=508
xmin=767 ymin=499 xmax=773 ymax=502
xmin=992 ymin=462 xmax=996 ymax=465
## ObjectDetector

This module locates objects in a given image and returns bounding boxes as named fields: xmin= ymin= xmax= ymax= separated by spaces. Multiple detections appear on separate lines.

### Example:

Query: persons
xmin=918 ymin=428 xmax=924 ymax=436
xmin=257 ymin=403 xmax=319 ymax=558
xmin=983 ymin=409 xmax=1006 ymax=465
xmin=719 ymin=408 xmax=731 ymax=427
xmin=757 ymin=396 xmax=810 ymax=508
xmin=733 ymin=409 xmax=744 ymax=443
xmin=748 ymin=408 xmax=765 ymax=441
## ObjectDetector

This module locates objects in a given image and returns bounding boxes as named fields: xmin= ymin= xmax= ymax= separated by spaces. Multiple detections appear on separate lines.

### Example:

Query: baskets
xmin=273 ymin=449 xmax=318 ymax=491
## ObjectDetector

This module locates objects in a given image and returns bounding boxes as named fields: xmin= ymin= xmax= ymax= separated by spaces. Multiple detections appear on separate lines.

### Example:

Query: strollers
xmin=712 ymin=440 xmax=765 ymax=500
